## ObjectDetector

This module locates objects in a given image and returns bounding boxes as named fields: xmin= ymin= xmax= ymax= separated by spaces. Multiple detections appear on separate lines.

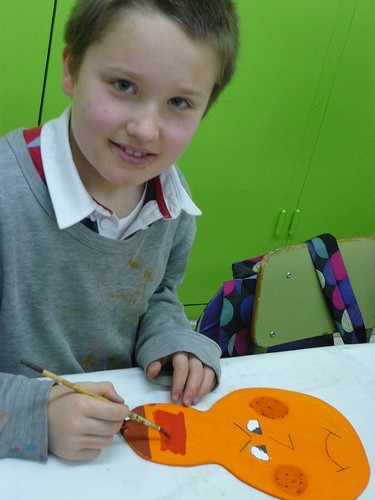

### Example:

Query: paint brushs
xmin=21 ymin=359 xmax=171 ymax=439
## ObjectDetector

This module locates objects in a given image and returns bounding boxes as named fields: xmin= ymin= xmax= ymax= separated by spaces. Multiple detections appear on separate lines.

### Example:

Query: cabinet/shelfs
xmin=39 ymin=0 xmax=375 ymax=305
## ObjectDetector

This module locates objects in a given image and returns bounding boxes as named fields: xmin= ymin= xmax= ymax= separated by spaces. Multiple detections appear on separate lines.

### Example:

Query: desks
xmin=0 ymin=342 xmax=375 ymax=500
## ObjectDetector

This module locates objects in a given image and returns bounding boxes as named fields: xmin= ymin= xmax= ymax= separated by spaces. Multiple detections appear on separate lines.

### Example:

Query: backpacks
xmin=193 ymin=231 xmax=368 ymax=361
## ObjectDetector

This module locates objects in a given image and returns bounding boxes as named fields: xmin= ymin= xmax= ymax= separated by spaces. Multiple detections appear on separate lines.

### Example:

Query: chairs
xmin=252 ymin=237 xmax=375 ymax=353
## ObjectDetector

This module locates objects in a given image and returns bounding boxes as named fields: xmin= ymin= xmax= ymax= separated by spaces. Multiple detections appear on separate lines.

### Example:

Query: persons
xmin=0 ymin=0 xmax=240 ymax=464
